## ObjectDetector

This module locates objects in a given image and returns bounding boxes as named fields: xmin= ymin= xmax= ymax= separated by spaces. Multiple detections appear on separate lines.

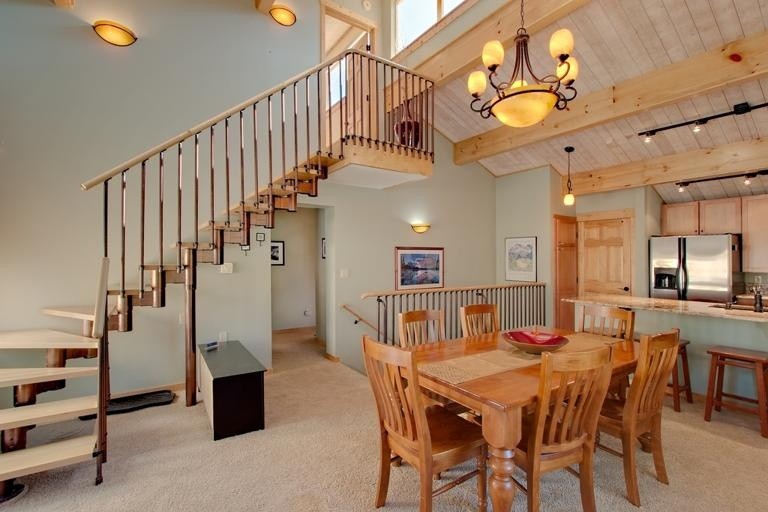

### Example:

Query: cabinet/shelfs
xmin=740 ymin=196 xmax=768 ymax=274
xmin=660 ymin=193 xmax=742 ymax=234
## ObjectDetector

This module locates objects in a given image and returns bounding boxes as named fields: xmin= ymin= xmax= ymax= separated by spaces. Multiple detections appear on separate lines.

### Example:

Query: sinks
xmin=708 ymin=305 xmax=755 ymax=311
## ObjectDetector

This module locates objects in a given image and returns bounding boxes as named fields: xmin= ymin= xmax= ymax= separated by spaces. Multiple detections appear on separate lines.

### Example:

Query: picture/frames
xmin=504 ymin=236 xmax=537 ymax=283
xmin=271 ymin=241 xmax=285 ymax=266
xmin=394 ymin=246 xmax=444 ymax=291
xmin=322 ymin=238 xmax=327 ymax=260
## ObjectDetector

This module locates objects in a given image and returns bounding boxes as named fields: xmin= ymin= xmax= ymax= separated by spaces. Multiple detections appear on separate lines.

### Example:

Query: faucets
xmin=726 ymin=301 xmax=736 ymax=309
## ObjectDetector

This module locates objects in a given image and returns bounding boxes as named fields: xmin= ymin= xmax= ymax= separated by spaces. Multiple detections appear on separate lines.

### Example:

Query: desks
xmin=199 ymin=341 xmax=266 ymax=440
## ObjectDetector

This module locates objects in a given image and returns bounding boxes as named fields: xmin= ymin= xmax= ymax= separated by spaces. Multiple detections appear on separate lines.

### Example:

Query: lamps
xmin=93 ymin=20 xmax=138 ymax=47
xmin=270 ymin=7 xmax=296 ymax=25
xmin=564 ymin=146 xmax=575 ymax=206
xmin=411 ymin=224 xmax=430 ymax=234
xmin=468 ymin=28 xmax=577 ymax=127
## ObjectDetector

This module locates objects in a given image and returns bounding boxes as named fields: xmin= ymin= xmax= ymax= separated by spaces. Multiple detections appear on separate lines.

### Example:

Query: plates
xmin=501 ymin=328 xmax=570 ymax=355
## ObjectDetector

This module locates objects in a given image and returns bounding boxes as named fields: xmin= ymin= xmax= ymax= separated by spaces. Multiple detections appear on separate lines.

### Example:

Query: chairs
xmin=461 ymin=304 xmax=498 ymax=338
xmin=561 ymin=329 xmax=681 ymax=508
xmin=361 ymin=335 xmax=488 ymax=512
xmin=578 ymin=305 xmax=635 ymax=401
xmin=475 ymin=346 xmax=613 ymax=512
xmin=401 ymin=309 xmax=473 ymax=415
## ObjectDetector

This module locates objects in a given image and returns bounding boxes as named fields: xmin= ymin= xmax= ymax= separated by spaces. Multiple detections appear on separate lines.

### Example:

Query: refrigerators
xmin=649 ymin=232 xmax=743 ymax=303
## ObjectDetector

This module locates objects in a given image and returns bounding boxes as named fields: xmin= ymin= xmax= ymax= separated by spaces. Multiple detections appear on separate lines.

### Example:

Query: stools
xmin=704 ymin=345 xmax=768 ymax=439
xmin=627 ymin=333 xmax=693 ymax=413
